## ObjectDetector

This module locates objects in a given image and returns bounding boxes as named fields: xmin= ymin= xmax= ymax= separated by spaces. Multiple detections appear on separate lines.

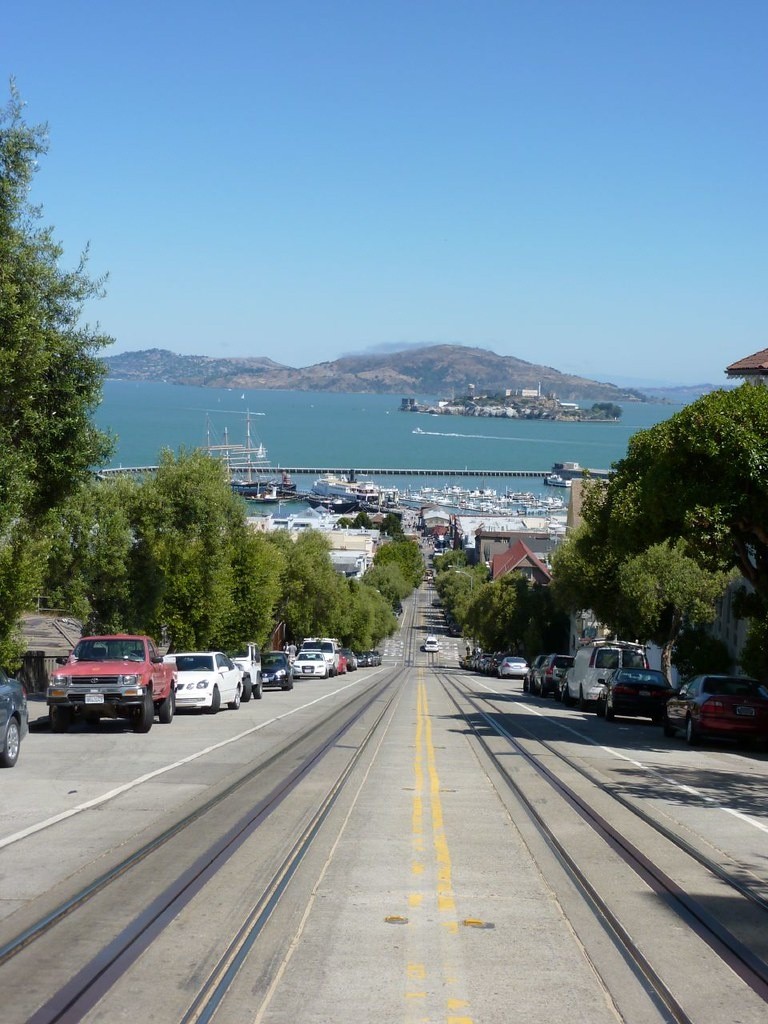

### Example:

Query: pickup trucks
xmin=46 ymin=634 xmax=179 ymax=734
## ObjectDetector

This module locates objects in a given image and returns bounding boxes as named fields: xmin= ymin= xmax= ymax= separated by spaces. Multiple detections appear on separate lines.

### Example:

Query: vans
xmin=568 ymin=639 xmax=652 ymax=712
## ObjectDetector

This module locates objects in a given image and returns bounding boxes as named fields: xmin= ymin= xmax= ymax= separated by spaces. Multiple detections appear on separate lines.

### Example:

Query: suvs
xmin=535 ymin=653 xmax=576 ymax=700
xmin=206 ymin=641 xmax=263 ymax=701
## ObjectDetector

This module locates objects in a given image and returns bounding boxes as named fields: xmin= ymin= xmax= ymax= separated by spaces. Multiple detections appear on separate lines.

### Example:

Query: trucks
xmin=300 ymin=638 xmax=340 ymax=677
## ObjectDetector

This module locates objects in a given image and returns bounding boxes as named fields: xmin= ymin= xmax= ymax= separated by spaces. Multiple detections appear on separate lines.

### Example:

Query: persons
xmin=471 ymin=649 xmax=477 ymax=656
xmin=282 ymin=640 xmax=298 ymax=659
xmin=466 ymin=645 xmax=470 ymax=655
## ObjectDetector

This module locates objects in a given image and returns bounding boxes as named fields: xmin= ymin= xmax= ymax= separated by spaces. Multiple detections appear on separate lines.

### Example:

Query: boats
xmin=197 ymin=408 xmax=566 ymax=521
xmin=545 ymin=474 xmax=572 ymax=489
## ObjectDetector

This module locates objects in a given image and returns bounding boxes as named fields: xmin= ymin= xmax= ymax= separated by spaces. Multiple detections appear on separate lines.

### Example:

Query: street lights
xmin=456 ymin=571 xmax=474 ymax=592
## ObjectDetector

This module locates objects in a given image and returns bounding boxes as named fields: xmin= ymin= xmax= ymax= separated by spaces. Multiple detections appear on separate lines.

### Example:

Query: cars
xmin=160 ymin=652 xmax=244 ymax=715
xmin=257 ymin=650 xmax=294 ymax=691
xmin=424 ymin=637 xmax=441 ymax=652
xmin=499 ymin=656 xmax=530 ymax=679
xmin=0 ymin=667 xmax=29 ymax=768
xmin=460 ymin=651 xmax=502 ymax=677
xmin=293 ymin=651 xmax=329 ymax=680
xmin=523 ymin=655 xmax=547 ymax=695
xmin=555 ymin=657 xmax=574 ymax=704
xmin=665 ymin=674 xmax=768 ymax=747
xmin=432 ymin=598 xmax=464 ymax=638
xmin=339 ymin=647 xmax=382 ymax=675
xmin=597 ymin=667 xmax=674 ymax=724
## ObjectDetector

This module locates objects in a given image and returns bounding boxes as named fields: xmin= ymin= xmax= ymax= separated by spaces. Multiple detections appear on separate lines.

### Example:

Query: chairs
xmin=275 ymin=659 xmax=282 ymax=663
xmin=130 ymin=650 xmax=144 ymax=660
xmin=315 ymin=655 xmax=322 ymax=660
xmin=89 ymin=647 xmax=106 ymax=660
xmin=185 ymin=661 xmax=197 ymax=670
